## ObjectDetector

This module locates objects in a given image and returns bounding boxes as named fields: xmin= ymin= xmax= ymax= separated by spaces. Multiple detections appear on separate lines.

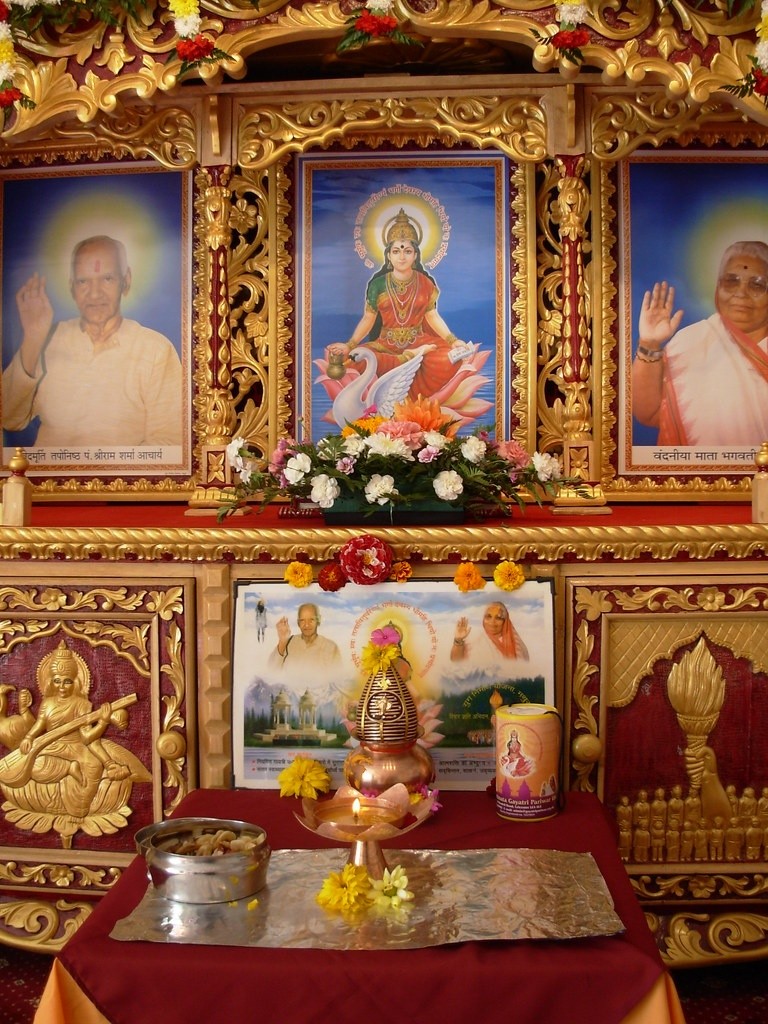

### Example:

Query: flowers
xmin=0 ymin=0 xmax=153 ymax=81
xmin=0 ymin=79 xmax=36 ymax=128
xmin=163 ymin=0 xmax=238 ymax=79
xmin=357 ymin=626 xmax=402 ymax=676
xmin=368 ymin=865 xmax=415 ymax=908
xmin=215 ymin=392 xmax=597 ymax=526
xmin=313 ymin=863 xmax=371 ymax=912
xmin=716 ymin=0 xmax=768 ymax=111
xmin=283 ymin=534 xmax=526 ymax=592
xmin=335 ymin=0 xmax=426 ymax=54
xmin=278 ymin=755 xmax=333 ymax=800
xmin=527 ymin=0 xmax=591 ymax=67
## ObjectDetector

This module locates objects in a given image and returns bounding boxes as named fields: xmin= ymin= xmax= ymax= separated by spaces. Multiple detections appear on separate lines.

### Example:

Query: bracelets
xmin=634 ymin=352 xmax=660 ymax=363
xmin=454 ymin=638 xmax=464 ymax=646
xmin=638 ymin=345 xmax=662 ymax=357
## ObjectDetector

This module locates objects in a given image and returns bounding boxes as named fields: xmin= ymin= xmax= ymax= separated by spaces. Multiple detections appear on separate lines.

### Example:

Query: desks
xmin=31 ymin=789 xmax=687 ymax=1024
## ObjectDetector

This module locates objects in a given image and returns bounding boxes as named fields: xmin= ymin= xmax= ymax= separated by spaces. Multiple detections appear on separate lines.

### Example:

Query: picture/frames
xmin=0 ymin=74 xmax=768 ymax=500
xmin=226 ymin=566 xmax=567 ymax=797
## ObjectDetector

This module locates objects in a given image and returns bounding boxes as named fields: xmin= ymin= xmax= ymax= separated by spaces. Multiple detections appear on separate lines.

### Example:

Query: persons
xmin=18 ymin=639 xmax=126 ymax=825
xmin=269 ymin=603 xmax=339 ymax=666
xmin=617 ymin=786 xmax=768 ymax=861
xmin=0 ymin=234 xmax=184 ymax=446
xmin=632 ymin=240 xmax=768 ymax=445
xmin=452 ymin=600 xmax=529 ymax=662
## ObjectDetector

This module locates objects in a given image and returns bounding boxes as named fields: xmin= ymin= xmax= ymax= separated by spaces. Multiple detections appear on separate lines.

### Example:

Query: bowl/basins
xmin=134 ymin=816 xmax=272 ymax=905
